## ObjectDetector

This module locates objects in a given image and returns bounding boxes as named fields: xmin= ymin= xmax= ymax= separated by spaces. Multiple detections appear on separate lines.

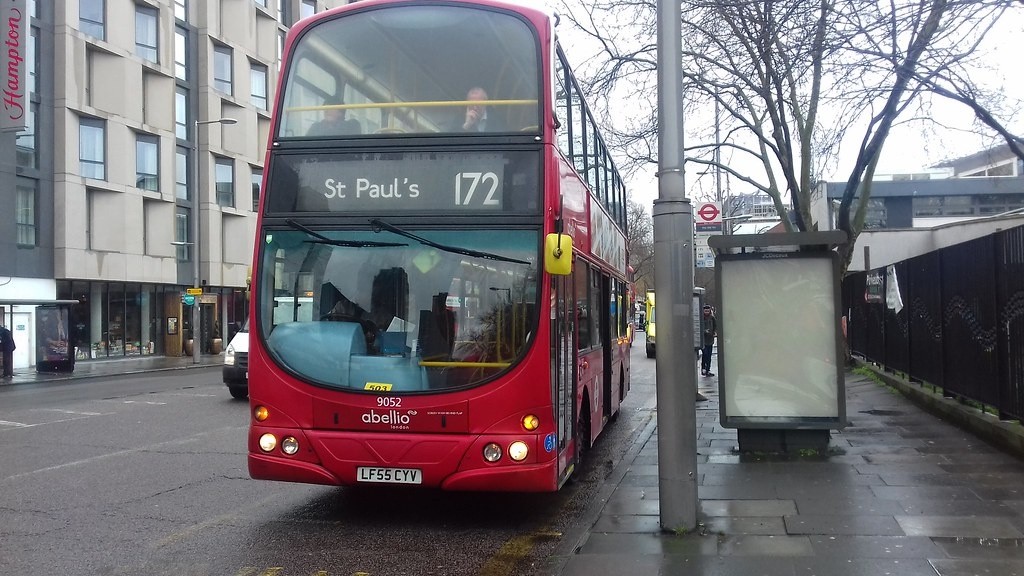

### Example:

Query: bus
xmin=247 ymin=0 xmax=635 ymax=493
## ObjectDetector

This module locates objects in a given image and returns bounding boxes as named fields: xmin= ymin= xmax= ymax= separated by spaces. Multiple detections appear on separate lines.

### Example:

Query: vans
xmin=223 ymin=297 xmax=313 ymax=398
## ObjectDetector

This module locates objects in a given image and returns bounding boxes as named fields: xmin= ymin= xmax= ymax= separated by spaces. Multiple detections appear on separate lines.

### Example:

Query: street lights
xmin=696 ymin=171 xmax=731 ymax=235
xmin=170 ymin=118 xmax=237 ymax=363
xmin=692 ymin=215 xmax=752 ymax=401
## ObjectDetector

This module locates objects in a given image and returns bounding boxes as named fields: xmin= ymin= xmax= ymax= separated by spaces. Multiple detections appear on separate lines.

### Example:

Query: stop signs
xmin=695 ymin=202 xmax=722 ymax=222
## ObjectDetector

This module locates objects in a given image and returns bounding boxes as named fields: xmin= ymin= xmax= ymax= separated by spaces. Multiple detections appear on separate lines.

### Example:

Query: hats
xmin=703 ymin=306 xmax=710 ymax=309
xmin=328 ymin=300 xmax=358 ymax=317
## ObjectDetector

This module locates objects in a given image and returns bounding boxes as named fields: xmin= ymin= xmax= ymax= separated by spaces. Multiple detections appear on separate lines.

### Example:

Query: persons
xmin=702 ymin=305 xmax=715 ymax=377
xmin=306 ymin=99 xmax=362 ymax=135
xmin=446 ymin=86 xmax=509 ymax=132
xmin=0 ymin=324 xmax=16 ymax=379
xmin=328 ymin=296 xmax=378 ymax=337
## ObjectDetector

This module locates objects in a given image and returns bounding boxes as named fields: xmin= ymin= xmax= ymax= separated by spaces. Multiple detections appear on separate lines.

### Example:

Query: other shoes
xmin=706 ymin=372 xmax=714 ymax=377
xmin=702 ymin=369 xmax=706 ymax=375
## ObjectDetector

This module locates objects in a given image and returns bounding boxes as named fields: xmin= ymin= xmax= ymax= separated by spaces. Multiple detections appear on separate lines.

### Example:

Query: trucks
xmin=645 ymin=289 xmax=656 ymax=357
